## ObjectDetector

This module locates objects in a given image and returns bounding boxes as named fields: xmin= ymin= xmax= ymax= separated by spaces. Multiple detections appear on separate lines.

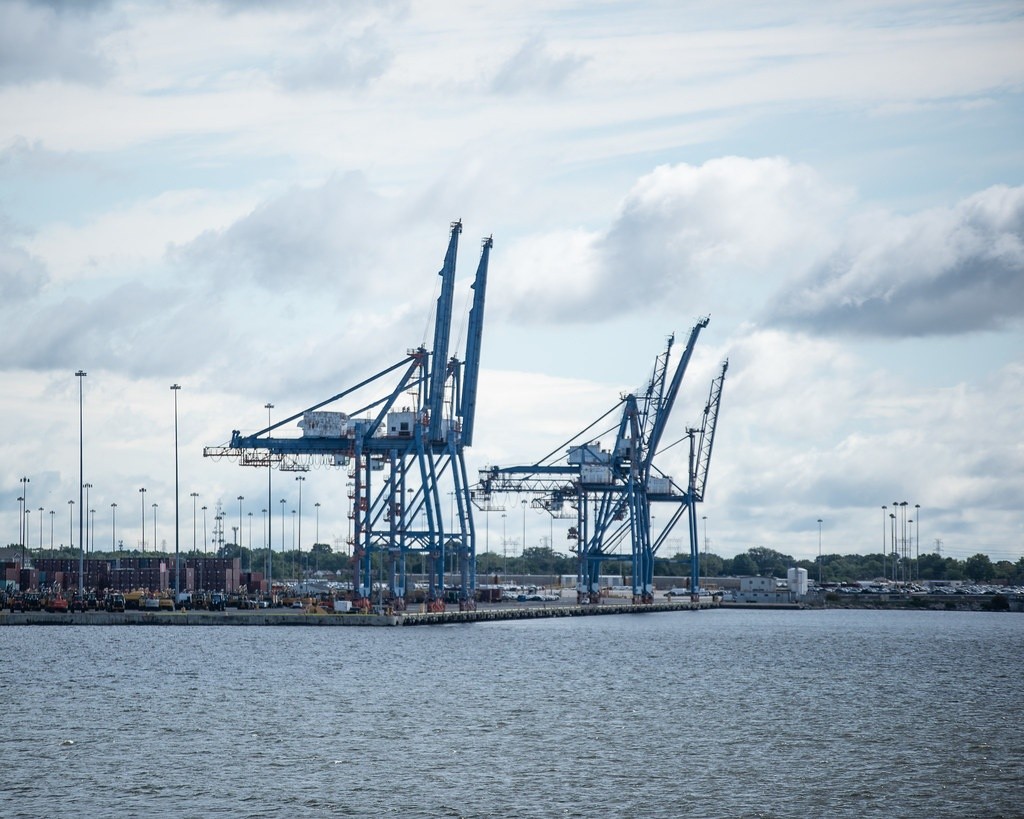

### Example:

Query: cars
xmin=806 ymin=584 xmax=1024 ymax=595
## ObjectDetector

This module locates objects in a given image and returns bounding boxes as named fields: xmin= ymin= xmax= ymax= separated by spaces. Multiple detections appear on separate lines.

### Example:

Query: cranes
xmin=204 ymin=217 xmax=732 ymax=605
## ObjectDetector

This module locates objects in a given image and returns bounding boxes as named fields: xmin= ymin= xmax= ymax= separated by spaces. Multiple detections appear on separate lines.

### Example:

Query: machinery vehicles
xmin=0 ymin=586 xmax=334 ymax=614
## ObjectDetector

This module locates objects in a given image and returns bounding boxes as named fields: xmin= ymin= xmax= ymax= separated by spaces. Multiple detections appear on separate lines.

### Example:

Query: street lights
xmin=446 ymin=492 xmax=457 ymax=588
xmin=502 ymin=513 xmax=508 ymax=582
xmin=291 ymin=510 xmax=297 ymax=583
xmin=651 ymin=515 xmax=655 ymax=549
xmin=189 ymin=493 xmax=239 ymax=592
xmin=248 ymin=512 xmax=254 ymax=572
xmin=14 ymin=476 xmax=158 ymax=592
xmin=236 ymin=494 xmax=245 ymax=571
xmin=280 ymin=499 xmax=287 ymax=583
xmin=295 ymin=475 xmax=306 ymax=584
xmin=408 ymin=488 xmax=415 ymax=586
xmin=314 ymin=503 xmax=320 ymax=585
xmin=701 ymin=516 xmax=708 ymax=591
xmin=261 ymin=509 xmax=268 ymax=581
xmin=882 ymin=500 xmax=921 ymax=589
xmin=521 ymin=499 xmax=528 ymax=586
xmin=169 ymin=382 xmax=183 ymax=607
xmin=73 ymin=370 xmax=88 ymax=600
xmin=817 ymin=519 xmax=824 ymax=587
xmin=265 ymin=402 xmax=274 ymax=595
